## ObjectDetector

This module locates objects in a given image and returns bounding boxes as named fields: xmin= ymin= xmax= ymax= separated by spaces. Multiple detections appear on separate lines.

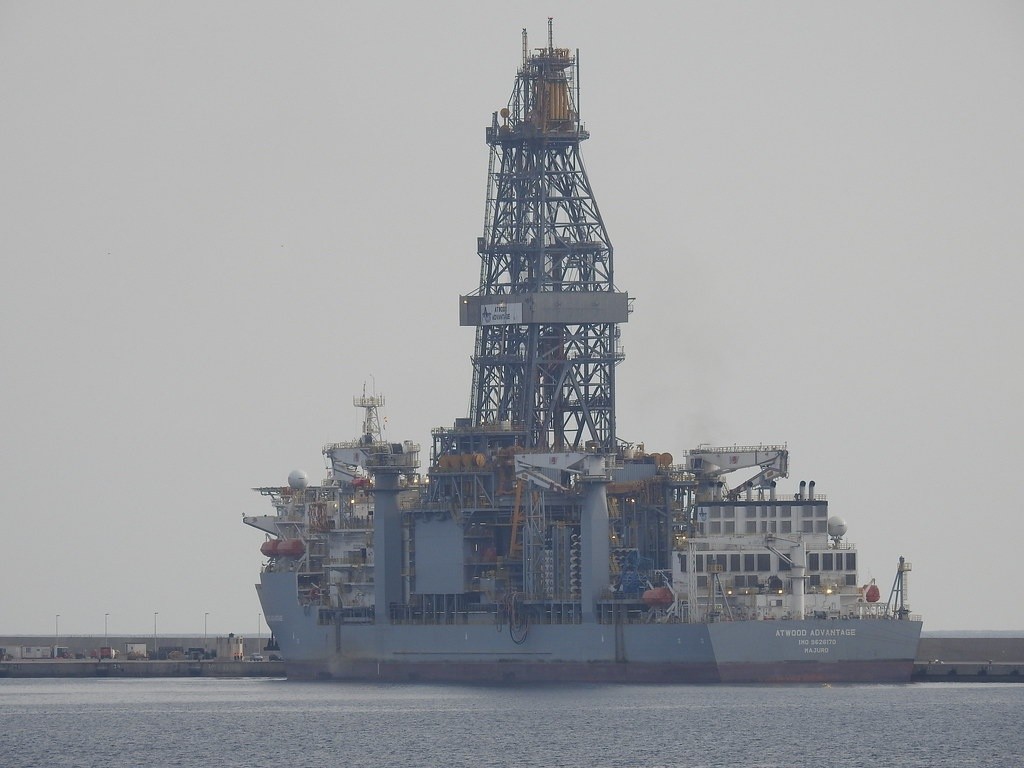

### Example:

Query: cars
xmin=251 ymin=653 xmax=263 ymax=660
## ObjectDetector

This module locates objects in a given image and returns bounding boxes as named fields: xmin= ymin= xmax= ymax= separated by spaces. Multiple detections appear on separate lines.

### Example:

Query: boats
xmin=244 ymin=16 xmax=922 ymax=685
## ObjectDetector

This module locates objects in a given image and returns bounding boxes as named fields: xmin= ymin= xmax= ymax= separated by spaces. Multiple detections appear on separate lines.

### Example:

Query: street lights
xmin=204 ymin=613 xmax=208 ymax=648
xmin=104 ymin=614 xmax=109 ymax=647
xmin=56 ymin=615 xmax=60 ymax=644
xmin=153 ymin=612 xmax=158 ymax=657
